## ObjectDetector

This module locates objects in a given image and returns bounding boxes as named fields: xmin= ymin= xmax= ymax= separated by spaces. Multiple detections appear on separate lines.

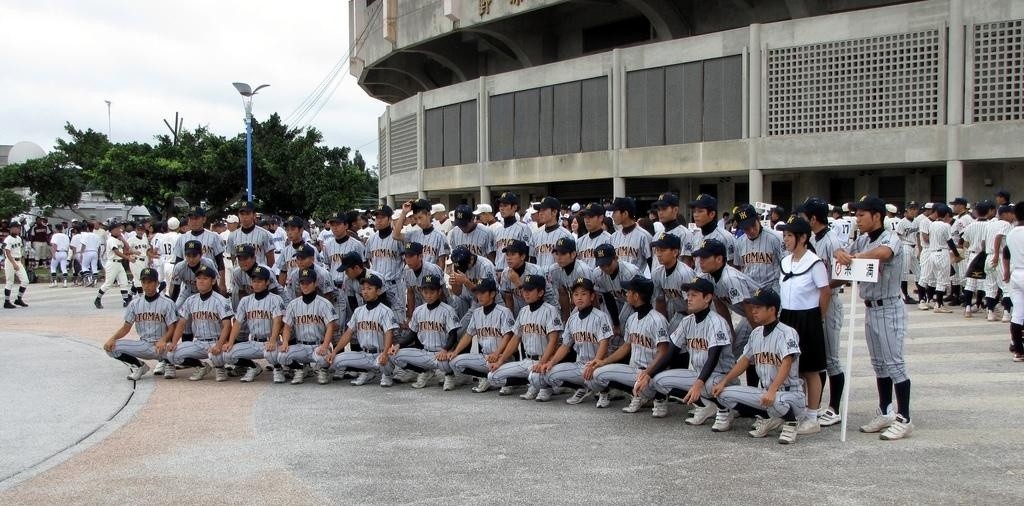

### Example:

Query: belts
xmin=364 ymin=349 xmax=378 ymax=353
xmin=526 ymin=353 xmax=541 ymax=360
xmin=135 ymin=257 xmax=144 ymax=261
xmin=253 ymin=338 xmax=267 ymax=342
xmin=298 ymin=340 xmax=317 ymax=345
xmin=197 ymin=336 xmax=220 ymax=343
xmin=864 ymin=298 xmax=884 ymax=308
xmin=113 ymin=259 xmax=124 ymax=262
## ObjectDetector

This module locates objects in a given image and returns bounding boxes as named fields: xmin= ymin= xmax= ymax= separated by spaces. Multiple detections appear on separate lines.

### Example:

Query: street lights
xmin=232 ymin=82 xmax=270 ymax=206
xmin=106 ymin=100 xmax=112 ymax=142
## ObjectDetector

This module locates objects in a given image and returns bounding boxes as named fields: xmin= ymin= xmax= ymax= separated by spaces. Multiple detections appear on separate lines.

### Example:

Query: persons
xmin=829 ymin=190 xmax=1024 ymax=361
xmin=834 ymin=195 xmax=915 ymax=438
xmin=104 ymin=191 xmax=845 ymax=435
xmin=711 ymin=289 xmax=805 ymax=444
xmin=1 ymin=216 xmax=131 ymax=309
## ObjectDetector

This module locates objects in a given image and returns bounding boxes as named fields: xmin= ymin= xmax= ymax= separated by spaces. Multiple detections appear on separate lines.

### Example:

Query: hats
xmin=832 ymin=196 xmax=897 ymax=215
xmin=393 ymin=198 xmax=445 ymax=218
xmin=691 ymin=238 xmax=726 ymax=257
xmin=8 ymin=215 xmax=188 ymax=233
xmin=260 ymin=215 xmax=303 ymax=228
xmin=140 ymin=268 xmax=159 ymax=281
xmin=496 ymin=191 xmax=519 ymax=205
xmin=594 ymin=244 xmax=616 ymax=267
xmin=371 ymin=206 xmax=392 ymax=218
xmin=238 ymin=201 xmax=254 ymax=211
xmin=222 ymin=215 xmax=240 ymax=223
xmin=502 ymin=239 xmax=527 ymax=255
xmin=681 ymin=277 xmax=714 ymax=295
xmin=471 ymin=279 xmax=496 ymax=291
xmin=743 ymin=288 xmax=781 ymax=308
xmin=906 ymin=191 xmax=1017 ymax=217
xmin=451 ymin=246 xmax=470 ymax=274
xmin=454 ymin=204 xmax=493 ymax=225
xmin=187 ymin=206 xmax=204 ymax=217
xmin=651 ymin=193 xmax=830 ymax=231
xmin=551 ymin=237 xmax=576 ymax=253
xmin=534 ymin=196 xmax=636 ymax=216
xmin=651 ymin=233 xmax=680 ymax=249
xmin=518 ymin=275 xmax=546 ymax=290
xmin=620 ymin=274 xmax=654 ymax=297
xmin=571 ymin=278 xmax=594 ymax=294
xmin=399 ymin=241 xmax=424 ymax=255
xmin=421 ymin=274 xmax=441 ymax=291
xmin=184 ymin=240 xmax=382 ymax=289
xmin=328 ymin=212 xmax=345 ymax=223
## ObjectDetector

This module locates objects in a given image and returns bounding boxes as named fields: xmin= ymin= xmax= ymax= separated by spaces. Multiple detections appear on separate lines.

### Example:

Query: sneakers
xmin=214 ymin=367 xmax=227 ymax=381
xmin=164 ymin=364 xmax=176 ymax=379
xmin=520 ymin=385 xmax=538 ymax=400
xmin=228 ymin=366 xmax=244 ymax=377
xmin=903 ymin=289 xmax=1012 ymax=323
xmin=265 ymin=363 xmax=469 ymax=391
xmin=566 ymin=389 xmax=593 ymax=405
xmin=651 ymin=394 xmax=669 ymax=418
xmin=880 ymin=413 xmax=913 ymax=441
xmin=94 ymin=299 xmax=102 ymax=308
xmin=685 ymin=403 xmax=719 ymax=425
xmin=622 ymin=395 xmax=649 ymax=412
xmin=498 ymin=386 xmax=513 ymax=396
xmin=859 ymin=408 xmax=897 ymax=432
xmin=127 ymin=362 xmax=150 ymax=380
xmin=15 ymin=298 xmax=28 ymax=307
xmin=188 ymin=362 xmax=212 ymax=381
xmin=153 ymin=360 xmax=166 ymax=375
xmin=535 ymin=388 xmax=554 ymax=401
xmin=595 ymin=388 xmax=612 ymax=409
xmin=48 ymin=277 xmax=98 ymax=288
xmin=4 ymin=301 xmax=15 ymax=307
xmin=749 ymin=399 xmax=843 ymax=444
xmin=240 ymin=363 xmax=262 ymax=382
xmin=472 ymin=377 xmax=491 ymax=393
xmin=712 ymin=408 xmax=734 ymax=432
